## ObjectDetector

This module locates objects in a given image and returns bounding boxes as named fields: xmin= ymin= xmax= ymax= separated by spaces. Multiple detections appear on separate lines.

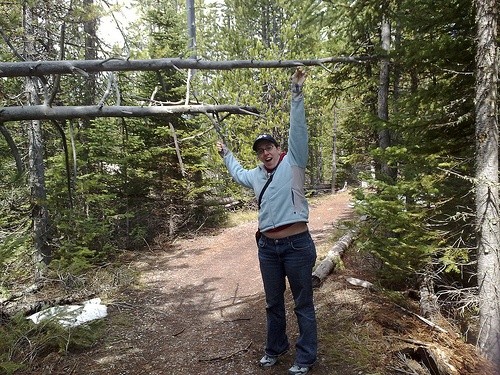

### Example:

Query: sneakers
xmin=287 ymin=361 xmax=314 ymax=374
xmin=257 ymin=353 xmax=282 ymax=367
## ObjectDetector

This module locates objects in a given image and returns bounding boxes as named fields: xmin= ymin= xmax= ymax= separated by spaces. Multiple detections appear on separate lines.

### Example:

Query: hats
xmin=252 ymin=134 xmax=279 ymax=149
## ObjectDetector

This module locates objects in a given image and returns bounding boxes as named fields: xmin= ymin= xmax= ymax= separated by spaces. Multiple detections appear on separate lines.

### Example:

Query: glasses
xmin=255 ymin=144 xmax=276 ymax=155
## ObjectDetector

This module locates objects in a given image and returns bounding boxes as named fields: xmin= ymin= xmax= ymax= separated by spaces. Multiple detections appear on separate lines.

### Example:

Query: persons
xmin=216 ymin=66 xmax=317 ymax=375
